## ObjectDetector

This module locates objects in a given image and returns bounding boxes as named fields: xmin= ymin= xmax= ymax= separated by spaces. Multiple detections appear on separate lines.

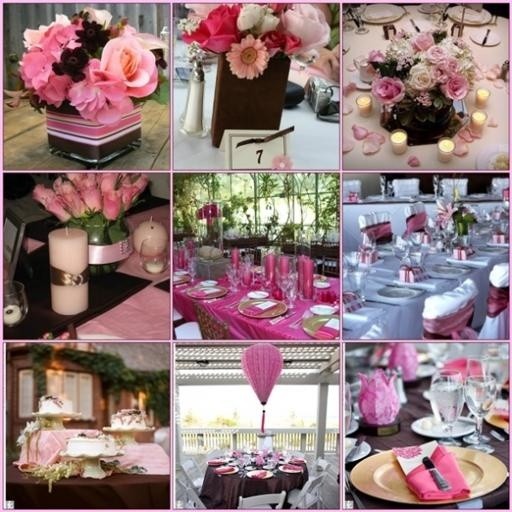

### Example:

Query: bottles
xmin=178 ymin=61 xmax=207 ymax=136
xmin=384 ymin=366 xmax=407 ymax=404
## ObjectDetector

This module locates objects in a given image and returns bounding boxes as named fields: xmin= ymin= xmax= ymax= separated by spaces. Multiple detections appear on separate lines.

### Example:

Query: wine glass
xmin=226 ymin=254 xmax=299 ymax=309
xmin=431 ymin=369 xmax=465 ymax=445
xmin=464 ymin=348 xmax=509 ymax=453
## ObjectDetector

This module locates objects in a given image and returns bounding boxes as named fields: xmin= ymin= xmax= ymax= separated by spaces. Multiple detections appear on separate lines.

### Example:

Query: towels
xmin=312 ymin=316 xmax=339 ymax=341
xmin=252 ymin=471 xmax=268 ymax=479
xmin=211 ymin=465 xmax=234 ymax=474
xmin=49 ymin=283 xmax=172 ymax=343
xmin=405 ymin=444 xmax=472 ymax=501
xmin=20 ymin=236 xmax=45 ymax=254
xmin=190 ymin=286 xmax=226 ymax=299
xmin=113 ymin=204 xmax=170 ymax=285
xmin=243 ymin=299 xmax=280 ymax=318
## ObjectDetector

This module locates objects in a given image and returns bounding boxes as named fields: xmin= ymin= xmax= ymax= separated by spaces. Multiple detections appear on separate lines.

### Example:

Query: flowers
xmin=25 ymin=172 xmax=157 ymax=224
xmin=360 ymin=22 xmax=479 ymax=113
xmin=16 ymin=6 xmax=167 ymax=131
xmin=176 ymin=3 xmax=333 ymax=83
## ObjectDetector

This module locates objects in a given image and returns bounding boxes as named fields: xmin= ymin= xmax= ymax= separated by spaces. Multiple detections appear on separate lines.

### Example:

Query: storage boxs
xmin=6 ymin=457 xmax=171 ymax=508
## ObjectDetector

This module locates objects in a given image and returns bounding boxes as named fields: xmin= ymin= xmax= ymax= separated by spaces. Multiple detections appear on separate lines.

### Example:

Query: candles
xmin=302 ymin=259 xmax=314 ymax=300
xmin=174 ymin=232 xmax=339 ymax=288
xmin=47 ymin=224 xmax=91 ymax=317
xmin=297 ymin=253 xmax=310 ymax=295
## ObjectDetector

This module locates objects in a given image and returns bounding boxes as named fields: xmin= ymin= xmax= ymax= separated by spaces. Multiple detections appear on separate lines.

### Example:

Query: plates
xmin=360 ymin=5 xmax=403 ymax=26
xmin=172 ymin=273 xmax=190 ymax=285
xmin=184 ymin=285 xmax=228 ymax=300
xmin=418 ymin=6 xmax=440 ymax=14
xmin=311 ymin=304 xmax=336 ymax=315
xmin=378 ymin=244 xmax=504 ymax=299
xmin=206 ymin=450 xmax=306 ymax=479
xmin=238 ymin=298 xmax=288 ymax=319
xmin=470 ymin=29 xmax=499 ymax=47
xmin=409 ymin=416 xmax=475 ymax=437
xmin=313 ymin=281 xmax=331 ymax=289
xmin=349 ymin=443 xmax=507 ymax=505
xmin=302 ymin=314 xmax=341 ymax=339
xmin=200 ymin=279 xmax=220 ymax=286
xmin=445 ymin=6 xmax=491 ymax=26
xmin=345 ymin=434 xmax=369 ymax=465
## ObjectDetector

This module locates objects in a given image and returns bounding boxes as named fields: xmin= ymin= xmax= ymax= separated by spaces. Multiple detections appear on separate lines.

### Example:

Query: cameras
xmin=307 ymin=79 xmax=333 ymax=113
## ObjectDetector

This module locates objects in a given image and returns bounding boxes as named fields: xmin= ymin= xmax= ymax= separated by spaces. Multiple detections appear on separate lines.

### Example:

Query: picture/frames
xmin=3 ymin=207 xmax=26 ymax=282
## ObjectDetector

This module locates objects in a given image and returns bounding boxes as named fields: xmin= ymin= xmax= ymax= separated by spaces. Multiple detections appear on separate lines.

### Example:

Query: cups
xmin=4 ymin=280 xmax=29 ymax=326
xmin=355 ymin=90 xmax=491 ymax=163
xmin=343 ymin=382 xmax=353 ymax=431
xmin=343 ymin=250 xmax=369 ymax=300
xmin=141 ymin=237 xmax=168 ymax=275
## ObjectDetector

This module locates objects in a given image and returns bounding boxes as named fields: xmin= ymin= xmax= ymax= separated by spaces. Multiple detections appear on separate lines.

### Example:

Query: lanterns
xmin=241 ymin=343 xmax=284 ymax=434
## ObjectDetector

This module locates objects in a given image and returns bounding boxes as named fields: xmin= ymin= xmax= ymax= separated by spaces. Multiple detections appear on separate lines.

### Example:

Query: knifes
xmin=480 ymin=29 xmax=490 ymax=45
xmin=409 ymin=19 xmax=421 ymax=34
xmin=422 ymin=454 xmax=450 ymax=489
xmin=346 ymin=434 xmax=366 ymax=460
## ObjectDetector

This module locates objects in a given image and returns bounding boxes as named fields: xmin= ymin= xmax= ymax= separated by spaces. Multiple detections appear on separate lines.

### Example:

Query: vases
xmin=59 ymin=208 xmax=135 ymax=279
xmin=39 ymin=93 xmax=147 ymax=169
xmin=202 ymin=41 xmax=294 ymax=153
xmin=373 ymin=88 xmax=460 ymax=143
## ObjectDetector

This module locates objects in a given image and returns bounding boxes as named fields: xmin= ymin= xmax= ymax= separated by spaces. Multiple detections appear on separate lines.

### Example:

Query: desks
xmin=4 ymin=99 xmax=173 ymax=172
xmin=18 ymin=423 xmax=168 ymax=476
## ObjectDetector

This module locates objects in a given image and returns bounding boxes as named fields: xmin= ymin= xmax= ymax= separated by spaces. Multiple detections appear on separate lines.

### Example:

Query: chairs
xmin=418 ymin=276 xmax=480 ymax=341
xmin=237 ymin=489 xmax=287 ymax=510
xmin=285 ymin=449 xmax=340 ymax=510
xmin=343 ymin=177 xmax=510 ymax=249
xmin=177 ymin=448 xmax=227 ymax=509
xmin=472 ymin=262 xmax=509 ymax=341
